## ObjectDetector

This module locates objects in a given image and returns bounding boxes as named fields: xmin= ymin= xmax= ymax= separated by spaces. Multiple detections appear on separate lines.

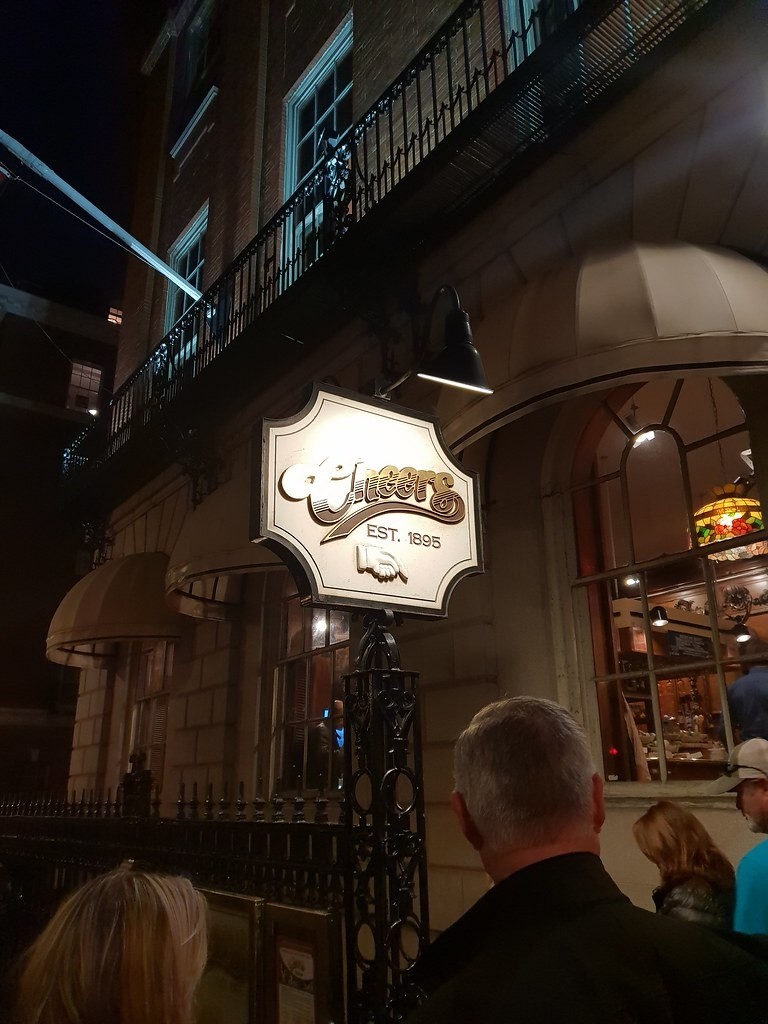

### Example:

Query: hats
xmin=706 ymin=737 xmax=768 ymax=794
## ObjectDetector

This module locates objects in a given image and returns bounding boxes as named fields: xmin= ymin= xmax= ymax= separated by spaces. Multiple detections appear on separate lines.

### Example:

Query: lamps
xmin=685 ymin=376 xmax=768 ymax=562
xmin=731 ymin=624 xmax=751 ymax=643
xmin=77 ymin=524 xmax=113 ymax=564
xmin=376 ymin=285 xmax=495 ymax=401
xmin=650 ymin=606 xmax=668 ymax=626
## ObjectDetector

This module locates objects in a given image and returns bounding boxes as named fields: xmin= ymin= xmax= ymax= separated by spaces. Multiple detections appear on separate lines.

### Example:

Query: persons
xmin=705 ymin=736 xmax=768 ymax=935
xmin=631 ymin=800 xmax=735 ymax=933
xmin=717 ymin=639 xmax=768 ymax=752
xmin=0 ymin=866 xmax=212 ymax=1024
xmin=388 ymin=697 xmax=768 ymax=1023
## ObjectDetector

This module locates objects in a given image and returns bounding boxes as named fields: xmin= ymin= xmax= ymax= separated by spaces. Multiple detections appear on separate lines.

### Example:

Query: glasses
xmin=722 ymin=764 xmax=768 ymax=778
